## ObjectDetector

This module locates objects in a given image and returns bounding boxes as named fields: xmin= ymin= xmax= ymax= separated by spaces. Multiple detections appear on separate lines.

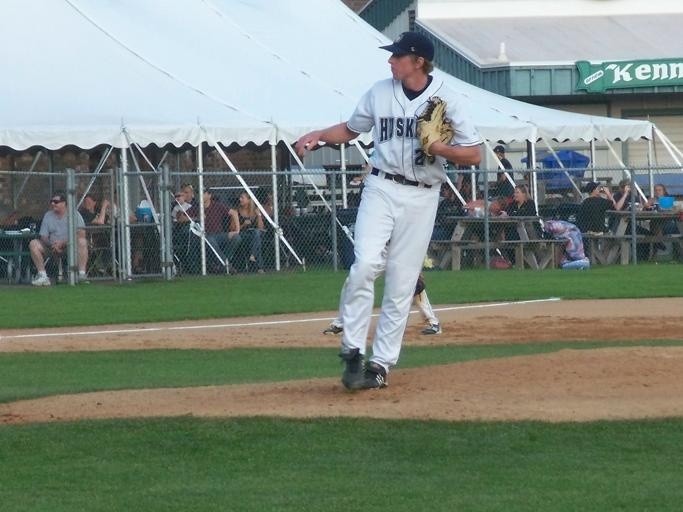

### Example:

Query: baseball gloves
xmin=414 ymin=96 xmax=453 ymax=156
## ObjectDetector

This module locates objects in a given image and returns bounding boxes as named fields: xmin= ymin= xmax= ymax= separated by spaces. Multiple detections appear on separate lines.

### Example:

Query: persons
xmin=173 ymin=183 xmax=276 ymax=274
xmin=293 ymin=32 xmax=485 ymax=390
xmin=320 ymin=146 xmax=443 ymax=337
xmin=432 ymin=147 xmax=682 ymax=262
xmin=28 ymin=194 xmax=141 ymax=285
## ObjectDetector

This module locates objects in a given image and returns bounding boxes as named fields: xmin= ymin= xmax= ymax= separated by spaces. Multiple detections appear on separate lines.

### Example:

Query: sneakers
xmin=323 ymin=324 xmax=342 ymax=335
xmin=78 ymin=274 xmax=89 ymax=284
xmin=339 ymin=348 xmax=385 ymax=390
xmin=32 ymin=274 xmax=51 ymax=286
xmin=422 ymin=322 xmax=442 ymax=335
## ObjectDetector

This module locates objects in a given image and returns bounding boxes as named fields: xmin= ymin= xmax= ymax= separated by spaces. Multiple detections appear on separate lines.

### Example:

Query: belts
xmin=369 ymin=167 xmax=431 ymax=188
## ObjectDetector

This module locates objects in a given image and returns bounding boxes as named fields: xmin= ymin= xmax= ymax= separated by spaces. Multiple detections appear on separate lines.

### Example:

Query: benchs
xmin=498 ymin=239 xmax=566 ymax=273
xmin=0 ymin=223 xmax=302 ymax=286
xmin=429 ymin=238 xmax=476 ymax=272
xmin=582 ymin=232 xmax=645 ymax=265
xmin=665 ymin=234 xmax=683 ymax=247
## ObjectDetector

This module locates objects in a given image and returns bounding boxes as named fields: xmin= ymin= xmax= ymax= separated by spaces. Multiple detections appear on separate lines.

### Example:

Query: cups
xmin=301 ymin=208 xmax=307 ymax=215
xmin=295 ymin=207 xmax=301 ymax=217
xmin=635 ymin=202 xmax=639 ymax=211
xmin=473 ymin=207 xmax=480 ymax=217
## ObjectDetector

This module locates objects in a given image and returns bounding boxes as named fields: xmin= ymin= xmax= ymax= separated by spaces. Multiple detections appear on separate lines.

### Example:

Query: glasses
xmin=50 ymin=199 xmax=64 ymax=203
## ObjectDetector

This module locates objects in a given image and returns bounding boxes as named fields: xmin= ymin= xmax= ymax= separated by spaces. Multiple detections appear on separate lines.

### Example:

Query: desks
xmin=606 ymin=209 xmax=683 ymax=266
xmin=437 ymin=215 xmax=541 ymax=271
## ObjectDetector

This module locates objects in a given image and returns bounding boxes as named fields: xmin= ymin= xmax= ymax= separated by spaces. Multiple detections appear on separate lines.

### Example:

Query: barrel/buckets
xmin=658 ymin=195 xmax=677 ymax=210
xmin=136 ymin=204 xmax=153 ymax=223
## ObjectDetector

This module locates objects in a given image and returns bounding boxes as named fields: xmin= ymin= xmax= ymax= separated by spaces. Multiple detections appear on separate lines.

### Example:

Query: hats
xmin=379 ymin=31 xmax=435 ymax=63
